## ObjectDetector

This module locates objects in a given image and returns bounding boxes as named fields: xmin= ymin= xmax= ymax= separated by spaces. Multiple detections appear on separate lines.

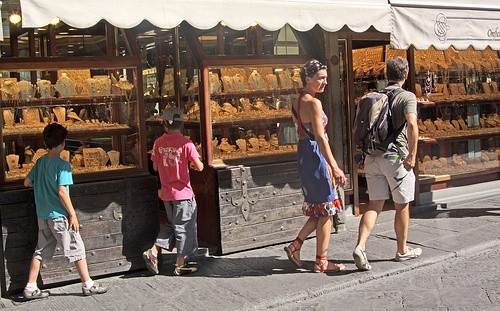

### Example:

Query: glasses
xmin=306 ymin=63 xmax=322 ymax=73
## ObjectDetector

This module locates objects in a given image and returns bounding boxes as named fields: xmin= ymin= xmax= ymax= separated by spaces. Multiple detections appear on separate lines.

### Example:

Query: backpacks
xmin=353 ymin=88 xmax=407 ymax=154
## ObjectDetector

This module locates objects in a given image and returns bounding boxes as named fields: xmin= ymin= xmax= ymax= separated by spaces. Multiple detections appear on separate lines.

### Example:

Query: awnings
xmin=20 ymin=0 xmax=500 ymax=54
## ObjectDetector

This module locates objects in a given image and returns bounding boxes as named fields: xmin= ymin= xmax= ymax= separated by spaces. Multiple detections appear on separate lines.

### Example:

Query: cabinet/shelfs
xmin=0 ymin=0 xmax=500 ymax=298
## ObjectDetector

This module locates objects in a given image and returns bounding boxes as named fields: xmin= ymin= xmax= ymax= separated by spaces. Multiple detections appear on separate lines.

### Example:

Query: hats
xmin=163 ymin=107 xmax=184 ymax=121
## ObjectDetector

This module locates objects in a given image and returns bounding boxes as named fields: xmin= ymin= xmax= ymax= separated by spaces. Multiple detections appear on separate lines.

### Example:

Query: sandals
xmin=284 ymin=237 xmax=306 ymax=268
xmin=313 ymin=255 xmax=345 ymax=273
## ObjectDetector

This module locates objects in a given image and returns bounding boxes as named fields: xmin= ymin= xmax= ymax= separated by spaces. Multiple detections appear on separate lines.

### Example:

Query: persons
xmin=142 ymin=106 xmax=204 ymax=275
xmin=353 ymin=56 xmax=422 ymax=271
xmin=284 ymin=59 xmax=347 ymax=273
xmin=22 ymin=122 xmax=108 ymax=300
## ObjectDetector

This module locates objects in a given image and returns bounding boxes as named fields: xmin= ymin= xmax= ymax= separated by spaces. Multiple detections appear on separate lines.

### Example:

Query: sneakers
xmin=353 ymin=246 xmax=372 ymax=270
xmin=82 ymin=282 xmax=110 ymax=296
xmin=174 ymin=262 xmax=198 ymax=276
xmin=142 ymin=249 xmax=159 ymax=274
xmin=23 ymin=289 xmax=50 ymax=300
xmin=395 ymin=246 xmax=422 ymax=261
